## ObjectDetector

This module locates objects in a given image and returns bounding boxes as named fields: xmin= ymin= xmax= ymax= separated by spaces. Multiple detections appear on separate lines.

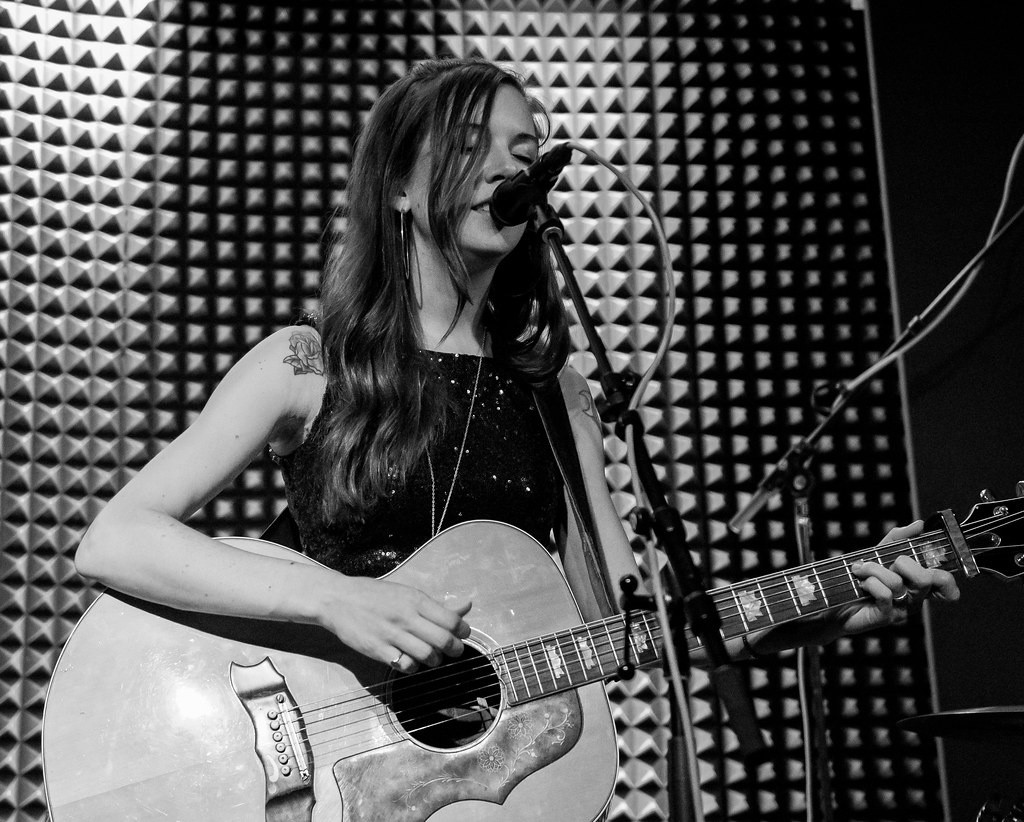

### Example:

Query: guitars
xmin=36 ymin=476 xmax=1024 ymax=822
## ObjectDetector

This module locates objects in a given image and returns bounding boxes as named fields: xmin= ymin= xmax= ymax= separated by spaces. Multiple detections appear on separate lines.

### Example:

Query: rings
xmin=893 ymin=585 xmax=909 ymax=604
xmin=391 ymin=652 xmax=404 ymax=669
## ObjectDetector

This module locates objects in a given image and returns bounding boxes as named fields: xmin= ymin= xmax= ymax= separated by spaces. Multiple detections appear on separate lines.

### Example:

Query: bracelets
xmin=741 ymin=635 xmax=762 ymax=661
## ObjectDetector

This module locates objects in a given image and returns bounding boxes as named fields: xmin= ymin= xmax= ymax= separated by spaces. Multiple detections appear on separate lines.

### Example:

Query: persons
xmin=76 ymin=57 xmax=960 ymax=676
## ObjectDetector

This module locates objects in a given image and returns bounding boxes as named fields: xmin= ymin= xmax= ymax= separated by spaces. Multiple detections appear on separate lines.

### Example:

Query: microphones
xmin=489 ymin=143 xmax=574 ymax=226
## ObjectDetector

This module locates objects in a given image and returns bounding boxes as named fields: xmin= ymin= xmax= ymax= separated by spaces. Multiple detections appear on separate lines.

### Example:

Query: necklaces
xmin=422 ymin=321 xmax=488 ymax=539
